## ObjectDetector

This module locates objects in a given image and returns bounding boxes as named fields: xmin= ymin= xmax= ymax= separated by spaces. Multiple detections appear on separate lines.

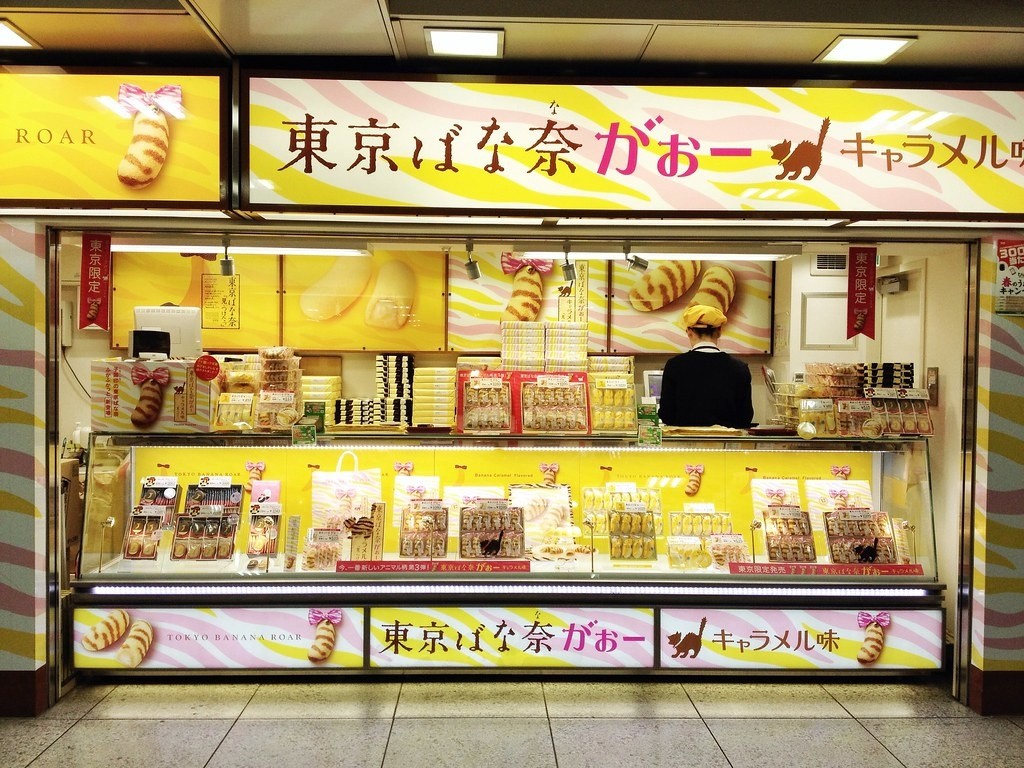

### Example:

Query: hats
xmin=683 ymin=305 xmax=727 ymax=328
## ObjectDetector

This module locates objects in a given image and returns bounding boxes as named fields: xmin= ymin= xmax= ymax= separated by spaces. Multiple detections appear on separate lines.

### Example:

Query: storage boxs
xmin=120 ymin=482 xmax=899 ymax=563
xmin=61 ymin=459 xmax=81 ymax=573
xmin=91 ymin=321 xmax=663 ymax=446
xmin=771 ymin=362 xmax=936 ymax=438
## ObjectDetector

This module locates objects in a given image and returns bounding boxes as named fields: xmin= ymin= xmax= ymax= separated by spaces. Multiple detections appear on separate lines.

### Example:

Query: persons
xmin=658 ymin=304 xmax=754 ymax=430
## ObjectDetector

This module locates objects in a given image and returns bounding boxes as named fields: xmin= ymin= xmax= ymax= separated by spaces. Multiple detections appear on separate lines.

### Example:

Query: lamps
xmin=220 ymin=233 xmax=234 ymax=276
xmin=812 ymin=35 xmax=918 ymax=64
xmin=464 ymin=243 xmax=480 ymax=281
xmin=562 ymin=239 xmax=576 ymax=281
xmin=110 ymin=233 xmax=374 ymax=256
xmin=423 ymin=27 xmax=505 ymax=59
xmin=622 ymin=244 xmax=648 ymax=273
xmin=512 ymin=244 xmax=802 ymax=261
xmin=0 ymin=18 xmax=43 ymax=50
